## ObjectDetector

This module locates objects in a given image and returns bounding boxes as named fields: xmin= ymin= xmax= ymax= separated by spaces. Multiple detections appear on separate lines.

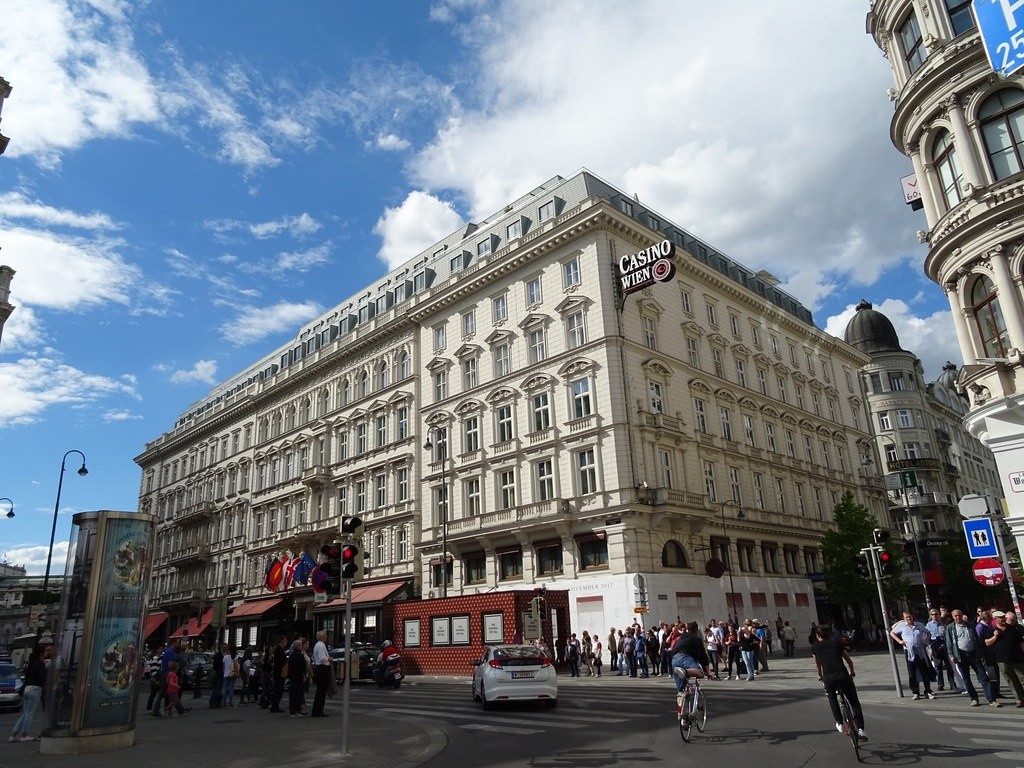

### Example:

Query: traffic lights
xmin=873 ymin=530 xmax=887 ymax=544
xmin=528 ymin=600 xmax=537 ymax=621
xmin=876 ymin=549 xmax=892 ymax=576
xmin=341 ymin=515 xmax=362 ymax=535
xmin=321 ymin=543 xmax=342 ymax=577
xmin=854 ymin=551 xmax=873 ymax=580
xmin=342 ymin=543 xmax=359 ymax=578
xmin=538 ymin=599 xmax=547 ymax=621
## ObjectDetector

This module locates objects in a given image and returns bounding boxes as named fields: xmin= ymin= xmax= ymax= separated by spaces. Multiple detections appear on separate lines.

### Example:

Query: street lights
xmin=722 ymin=499 xmax=746 ymax=629
xmin=422 ymin=426 xmax=448 ymax=598
xmin=859 ymin=434 xmax=932 ymax=621
xmin=41 ymin=450 xmax=89 ymax=607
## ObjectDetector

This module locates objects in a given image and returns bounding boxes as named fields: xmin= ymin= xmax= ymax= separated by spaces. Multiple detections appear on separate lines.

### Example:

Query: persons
xmin=7 ymin=634 xmax=311 ymax=741
xmin=535 ymin=618 xmax=883 ymax=679
xmin=373 ymin=640 xmax=400 ymax=688
xmin=312 ymin=631 xmax=333 ymax=715
xmin=671 ymin=620 xmax=716 ymax=720
xmin=811 ymin=626 xmax=870 ymax=742
xmin=889 ymin=605 xmax=1024 ymax=707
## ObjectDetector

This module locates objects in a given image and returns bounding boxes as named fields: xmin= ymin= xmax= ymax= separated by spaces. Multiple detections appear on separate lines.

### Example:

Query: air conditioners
xmin=427 ymin=588 xmax=441 ymax=599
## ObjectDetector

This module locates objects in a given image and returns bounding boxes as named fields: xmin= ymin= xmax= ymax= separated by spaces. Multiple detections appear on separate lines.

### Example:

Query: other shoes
xmin=1016 ymin=702 xmax=1024 ymax=708
xmin=681 ymin=721 xmax=690 ymax=732
xmin=961 ymin=690 xmax=968 ymax=694
xmin=990 ymin=701 xmax=1001 ymax=707
xmin=144 ymin=699 xmax=329 ymax=719
xmin=568 ymin=667 xmax=769 ymax=682
xmin=971 ymin=700 xmax=978 ymax=705
xmin=937 ymin=686 xmax=943 ymax=691
xmin=950 ymin=685 xmax=960 ymax=691
xmin=995 ymin=693 xmax=1003 ymax=698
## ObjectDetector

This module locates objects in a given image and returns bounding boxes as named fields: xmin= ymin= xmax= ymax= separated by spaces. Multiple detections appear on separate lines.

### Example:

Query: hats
xmin=718 ymin=621 xmax=724 ymax=625
xmin=992 ymin=611 xmax=1006 ymax=617
xmin=752 ymin=619 xmax=760 ymax=624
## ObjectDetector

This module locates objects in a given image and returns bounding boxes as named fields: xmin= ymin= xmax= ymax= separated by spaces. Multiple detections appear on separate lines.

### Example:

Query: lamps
xmin=962 ymin=183 xmax=977 ymax=201
xmin=1007 ymin=348 xmax=1022 ymax=365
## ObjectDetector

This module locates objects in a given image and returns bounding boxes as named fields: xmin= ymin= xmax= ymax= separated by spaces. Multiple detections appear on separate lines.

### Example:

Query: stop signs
xmin=972 ymin=557 xmax=1005 ymax=587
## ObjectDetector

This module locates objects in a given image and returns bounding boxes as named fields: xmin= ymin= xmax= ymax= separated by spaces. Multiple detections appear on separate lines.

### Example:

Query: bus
xmin=6 ymin=633 xmax=57 ymax=673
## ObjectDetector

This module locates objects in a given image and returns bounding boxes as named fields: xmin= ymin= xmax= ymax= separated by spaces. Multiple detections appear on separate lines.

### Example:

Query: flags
xmin=261 ymin=551 xmax=316 ymax=593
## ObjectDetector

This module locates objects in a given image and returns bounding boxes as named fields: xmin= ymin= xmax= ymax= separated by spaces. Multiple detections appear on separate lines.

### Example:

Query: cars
xmin=0 ymin=654 xmax=293 ymax=713
xmin=470 ymin=644 xmax=557 ymax=708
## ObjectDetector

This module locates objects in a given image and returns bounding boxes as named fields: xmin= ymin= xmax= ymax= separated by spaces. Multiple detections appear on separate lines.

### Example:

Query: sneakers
xmin=858 ymin=729 xmax=869 ymax=738
xmin=836 ymin=723 xmax=843 ymax=732
xmin=925 ymin=693 xmax=935 ymax=699
xmin=913 ymin=694 xmax=918 ymax=700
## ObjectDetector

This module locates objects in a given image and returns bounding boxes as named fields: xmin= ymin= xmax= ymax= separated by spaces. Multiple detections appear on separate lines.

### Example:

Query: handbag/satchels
xmin=581 ymin=652 xmax=586 ymax=663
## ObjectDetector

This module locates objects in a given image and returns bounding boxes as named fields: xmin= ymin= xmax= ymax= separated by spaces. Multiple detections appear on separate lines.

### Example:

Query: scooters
xmin=369 ymin=654 xmax=404 ymax=690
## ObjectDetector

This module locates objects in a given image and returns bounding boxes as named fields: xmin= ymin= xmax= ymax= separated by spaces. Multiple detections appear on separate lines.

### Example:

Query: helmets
xmin=383 ymin=640 xmax=392 ymax=648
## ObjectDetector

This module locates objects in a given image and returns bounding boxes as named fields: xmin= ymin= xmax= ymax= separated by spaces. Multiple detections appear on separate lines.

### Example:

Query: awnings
xmin=169 ymin=607 xmax=214 ymax=636
xmin=316 ymin=581 xmax=406 ymax=607
xmin=144 ymin=614 xmax=169 ymax=642
xmin=225 ymin=598 xmax=283 ymax=616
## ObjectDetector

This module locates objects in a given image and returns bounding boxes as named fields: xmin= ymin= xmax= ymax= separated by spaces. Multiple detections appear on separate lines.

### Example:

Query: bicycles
xmin=678 ymin=673 xmax=720 ymax=742
xmin=818 ymin=673 xmax=863 ymax=763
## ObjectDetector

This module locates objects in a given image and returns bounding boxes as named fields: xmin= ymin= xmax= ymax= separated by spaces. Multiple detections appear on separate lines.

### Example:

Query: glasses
xmin=977 ymin=611 xmax=983 ymax=613
